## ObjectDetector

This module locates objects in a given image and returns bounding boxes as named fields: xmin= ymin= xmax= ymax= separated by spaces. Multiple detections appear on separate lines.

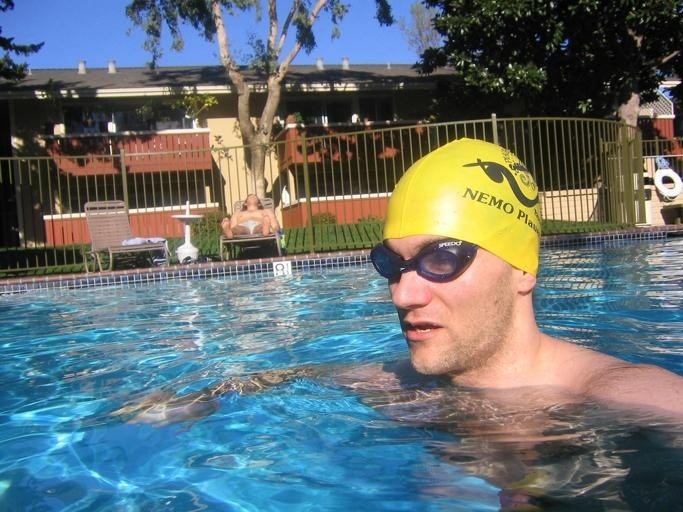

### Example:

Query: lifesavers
xmin=654 ymin=167 xmax=683 ymax=198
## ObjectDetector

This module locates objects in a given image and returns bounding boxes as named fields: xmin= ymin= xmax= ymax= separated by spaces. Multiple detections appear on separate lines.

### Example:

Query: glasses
xmin=370 ymin=238 xmax=478 ymax=283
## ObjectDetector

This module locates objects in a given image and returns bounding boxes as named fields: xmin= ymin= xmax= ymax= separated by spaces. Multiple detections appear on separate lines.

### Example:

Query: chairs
xmin=83 ymin=196 xmax=282 ymax=272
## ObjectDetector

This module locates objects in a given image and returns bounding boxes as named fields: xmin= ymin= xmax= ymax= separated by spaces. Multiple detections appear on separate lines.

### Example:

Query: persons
xmin=220 ymin=194 xmax=280 ymax=240
xmin=109 ymin=136 xmax=683 ymax=512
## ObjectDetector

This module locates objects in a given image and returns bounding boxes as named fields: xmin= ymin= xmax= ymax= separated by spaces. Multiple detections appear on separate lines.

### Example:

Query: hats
xmin=382 ymin=137 xmax=542 ymax=277
